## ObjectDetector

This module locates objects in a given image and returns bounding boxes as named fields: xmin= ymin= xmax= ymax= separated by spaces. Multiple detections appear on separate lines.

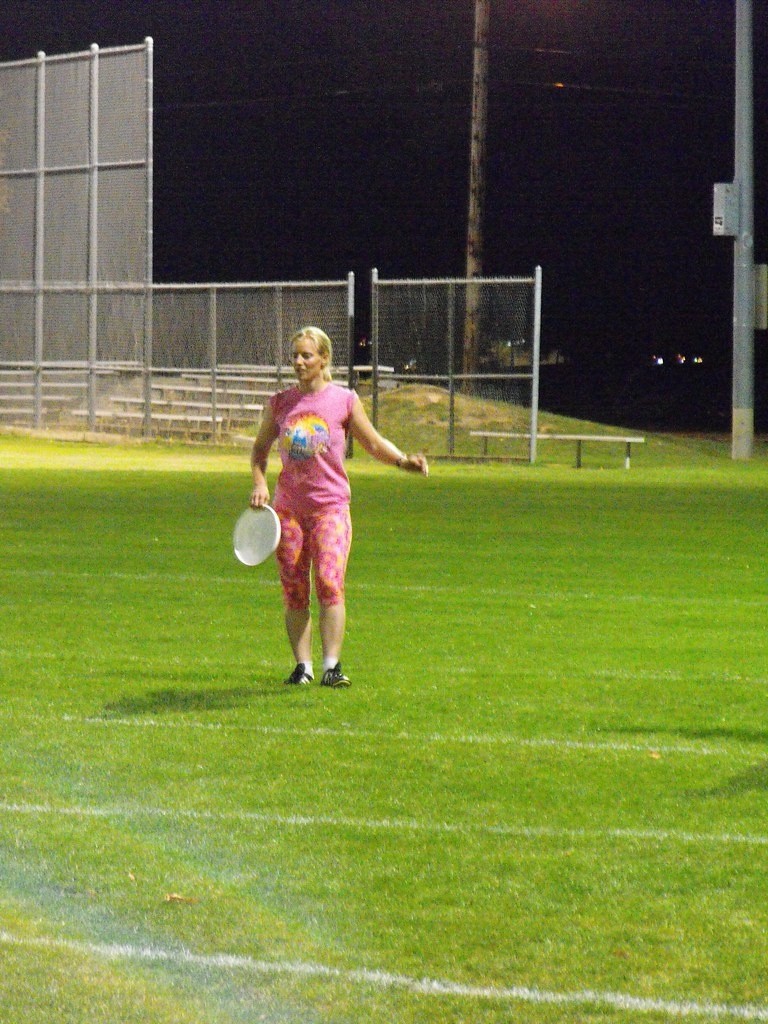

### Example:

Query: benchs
xmin=0 ymin=369 xmax=121 ymax=386
xmin=0 ymin=381 xmax=89 ymax=403
xmin=0 ymin=409 xmax=48 ymax=430
xmin=0 ymin=360 xmax=144 ymax=378
xmin=0 ymin=394 xmax=74 ymax=423
xmin=470 ymin=431 xmax=645 ymax=470
xmin=216 ymin=364 xmax=394 ymax=381
xmin=130 ymin=384 xmax=276 ymax=415
xmin=181 ymin=373 xmax=299 ymax=391
xmin=112 ymin=397 xmax=265 ymax=432
xmin=73 ymin=409 xmax=223 ymax=444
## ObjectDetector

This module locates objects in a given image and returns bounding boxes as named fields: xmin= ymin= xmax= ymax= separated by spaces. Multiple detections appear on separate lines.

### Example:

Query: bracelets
xmin=395 ymin=457 xmax=408 ymax=467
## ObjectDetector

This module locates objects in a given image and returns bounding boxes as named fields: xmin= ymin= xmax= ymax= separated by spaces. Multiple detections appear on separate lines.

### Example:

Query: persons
xmin=250 ymin=326 xmax=430 ymax=686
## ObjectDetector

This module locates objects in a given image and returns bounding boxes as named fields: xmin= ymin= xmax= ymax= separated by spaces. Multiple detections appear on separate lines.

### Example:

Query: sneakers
xmin=284 ymin=663 xmax=314 ymax=685
xmin=320 ymin=662 xmax=351 ymax=689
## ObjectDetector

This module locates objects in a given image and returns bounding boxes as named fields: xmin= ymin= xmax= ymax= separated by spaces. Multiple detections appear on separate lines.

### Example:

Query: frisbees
xmin=232 ymin=503 xmax=281 ymax=568
